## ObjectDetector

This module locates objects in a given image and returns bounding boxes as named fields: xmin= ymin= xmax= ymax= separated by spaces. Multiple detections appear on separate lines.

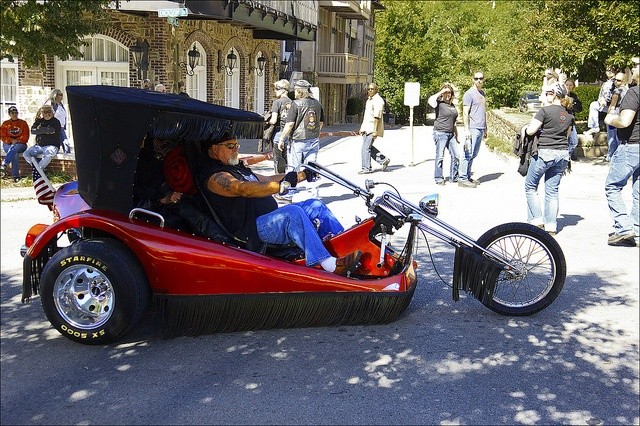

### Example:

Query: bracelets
xmin=281 ymin=138 xmax=286 ymax=142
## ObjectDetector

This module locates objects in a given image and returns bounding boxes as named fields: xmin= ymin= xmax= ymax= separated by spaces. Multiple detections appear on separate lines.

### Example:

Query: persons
xmin=133 ymin=133 xmax=267 ymax=255
xmin=155 ymin=84 xmax=165 ymax=92
xmin=458 ymin=71 xmax=488 ymax=187
xmin=23 ymin=105 xmax=61 ymax=171
xmin=584 ymin=65 xmax=616 ymax=161
xmin=198 ymin=127 xmax=362 ymax=276
xmin=44 ymin=89 xmax=72 ymax=153
xmin=278 ymin=79 xmax=324 ymax=203
xmin=540 ymin=72 xmax=561 ymax=106
xmin=142 ymin=79 xmax=151 ymax=90
xmin=428 ymin=85 xmax=460 ymax=185
xmin=604 ymin=57 xmax=640 ymax=247
xmin=268 ymin=79 xmax=293 ymax=174
xmin=0 ymin=106 xmax=30 ymax=182
xmin=606 ymin=73 xmax=629 ymax=162
xmin=564 ymin=78 xmax=582 ymax=161
xmin=357 ymin=82 xmax=390 ymax=173
xmin=524 ymin=82 xmax=572 ymax=235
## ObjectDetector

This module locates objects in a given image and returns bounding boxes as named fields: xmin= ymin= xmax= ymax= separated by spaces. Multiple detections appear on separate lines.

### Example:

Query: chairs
xmin=183 ymin=141 xmax=296 ymax=250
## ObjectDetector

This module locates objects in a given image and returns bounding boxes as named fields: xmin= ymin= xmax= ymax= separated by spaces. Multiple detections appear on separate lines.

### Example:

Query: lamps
xmin=255 ymin=53 xmax=267 ymax=77
xmin=220 ymin=47 xmax=237 ymax=76
xmin=278 ymin=56 xmax=289 ymax=76
xmin=187 ymin=45 xmax=200 ymax=76
xmin=130 ymin=38 xmax=145 ymax=80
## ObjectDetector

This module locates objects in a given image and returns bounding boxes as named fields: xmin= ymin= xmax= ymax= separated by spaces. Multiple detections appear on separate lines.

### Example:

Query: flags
xmin=32 ymin=164 xmax=54 ymax=211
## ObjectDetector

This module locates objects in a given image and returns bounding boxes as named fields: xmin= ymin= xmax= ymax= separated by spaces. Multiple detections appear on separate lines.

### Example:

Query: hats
xmin=208 ymin=129 xmax=236 ymax=145
xmin=294 ymin=79 xmax=312 ymax=89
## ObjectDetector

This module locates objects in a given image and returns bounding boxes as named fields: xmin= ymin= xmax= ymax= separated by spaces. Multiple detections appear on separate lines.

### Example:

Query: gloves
xmin=303 ymin=164 xmax=321 ymax=183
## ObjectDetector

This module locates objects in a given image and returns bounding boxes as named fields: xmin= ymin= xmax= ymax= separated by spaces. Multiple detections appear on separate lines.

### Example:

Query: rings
xmin=174 ymin=201 xmax=177 ymax=204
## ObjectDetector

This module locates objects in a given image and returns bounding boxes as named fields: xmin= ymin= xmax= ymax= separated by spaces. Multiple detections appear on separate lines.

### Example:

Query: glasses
xmin=474 ymin=76 xmax=484 ymax=81
xmin=368 ymin=87 xmax=374 ymax=90
xmin=275 ymin=87 xmax=281 ymax=90
xmin=57 ymin=92 xmax=63 ymax=95
xmin=10 ymin=111 xmax=19 ymax=114
xmin=218 ymin=141 xmax=241 ymax=149
xmin=42 ymin=109 xmax=52 ymax=113
xmin=615 ymin=78 xmax=622 ymax=82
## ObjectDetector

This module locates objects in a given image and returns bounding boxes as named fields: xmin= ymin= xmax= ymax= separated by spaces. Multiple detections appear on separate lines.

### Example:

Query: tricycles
xmin=20 ymin=84 xmax=567 ymax=345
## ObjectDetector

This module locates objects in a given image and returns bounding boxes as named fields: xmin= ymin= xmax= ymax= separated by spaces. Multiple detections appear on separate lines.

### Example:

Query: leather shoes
xmin=333 ymin=248 xmax=362 ymax=276
xmin=382 ymin=158 xmax=390 ymax=171
xmin=607 ymin=230 xmax=635 ymax=245
xmin=458 ymin=179 xmax=476 ymax=187
xmin=436 ymin=178 xmax=445 ymax=184
xmin=470 ymin=178 xmax=480 ymax=185
xmin=358 ymin=169 xmax=373 ymax=174
